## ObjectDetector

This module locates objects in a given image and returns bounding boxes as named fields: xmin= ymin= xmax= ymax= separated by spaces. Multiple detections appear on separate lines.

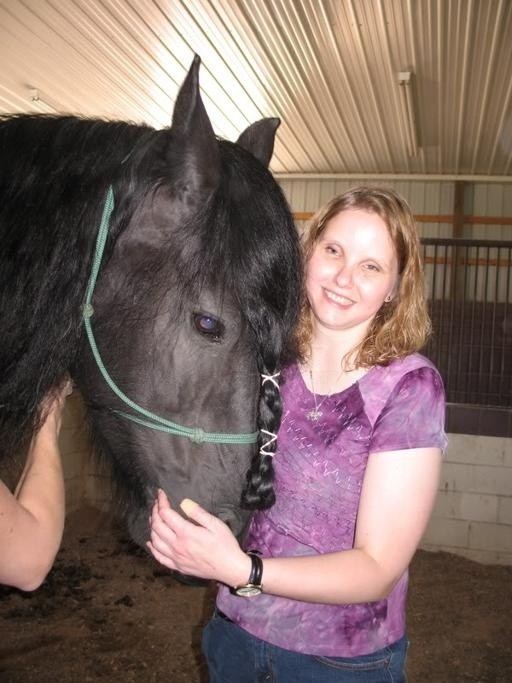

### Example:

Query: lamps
xmin=397 ymin=71 xmax=420 ymax=157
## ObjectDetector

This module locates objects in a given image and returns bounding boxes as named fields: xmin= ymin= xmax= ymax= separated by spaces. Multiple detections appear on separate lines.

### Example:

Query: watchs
xmin=226 ymin=546 xmax=270 ymax=602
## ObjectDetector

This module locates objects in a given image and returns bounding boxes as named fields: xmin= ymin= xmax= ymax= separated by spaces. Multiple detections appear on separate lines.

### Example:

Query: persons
xmin=1 ymin=371 xmax=78 ymax=598
xmin=139 ymin=185 xmax=453 ymax=681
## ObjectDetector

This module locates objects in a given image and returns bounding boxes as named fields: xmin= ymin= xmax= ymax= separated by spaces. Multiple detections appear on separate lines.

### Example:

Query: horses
xmin=0 ymin=52 xmax=306 ymax=590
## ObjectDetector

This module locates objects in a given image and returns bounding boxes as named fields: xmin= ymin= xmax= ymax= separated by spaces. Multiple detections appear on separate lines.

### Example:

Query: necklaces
xmin=303 ymin=346 xmax=353 ymax=424
